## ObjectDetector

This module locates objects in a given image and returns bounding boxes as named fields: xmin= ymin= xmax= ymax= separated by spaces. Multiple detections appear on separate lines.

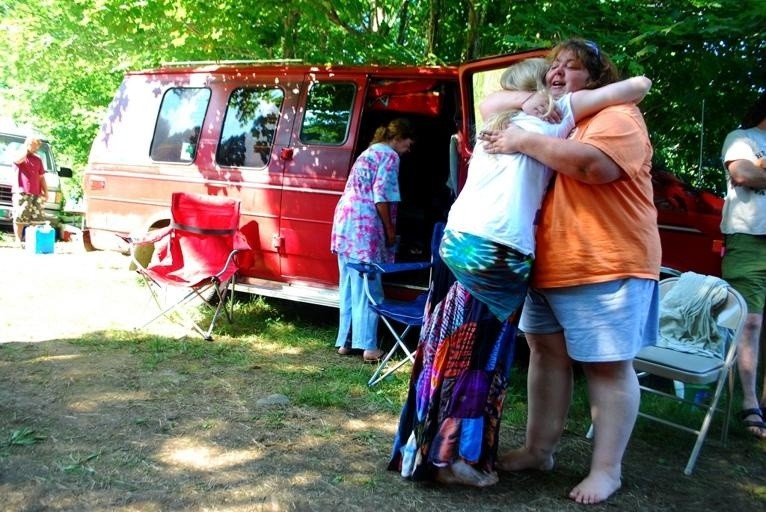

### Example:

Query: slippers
xmin=364 ymin=349 xmax=393 ymax=365
xmin=336 ymin=348 xmax=363 ymax=356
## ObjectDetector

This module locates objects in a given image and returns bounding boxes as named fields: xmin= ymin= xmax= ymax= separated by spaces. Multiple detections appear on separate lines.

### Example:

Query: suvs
xmin=0 ymin=132 xmax=72 ymax=241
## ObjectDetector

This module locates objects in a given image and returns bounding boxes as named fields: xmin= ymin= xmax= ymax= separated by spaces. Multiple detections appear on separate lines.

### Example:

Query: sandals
xmin=733 ymin=407 xmax=766 ymax=439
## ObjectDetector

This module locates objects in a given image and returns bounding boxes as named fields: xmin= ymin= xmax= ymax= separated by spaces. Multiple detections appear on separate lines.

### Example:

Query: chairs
xmin=124 ymin=190 xmax=253 ymax=342
xmin=346 ymin=222 xmax=447 ymax=388
xmin=585 ymin=273 xmax=749 ymax=475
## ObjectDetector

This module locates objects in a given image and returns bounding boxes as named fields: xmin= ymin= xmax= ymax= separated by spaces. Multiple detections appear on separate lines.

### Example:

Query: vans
xmin=80 ymin=47 xmax=728 ymax=376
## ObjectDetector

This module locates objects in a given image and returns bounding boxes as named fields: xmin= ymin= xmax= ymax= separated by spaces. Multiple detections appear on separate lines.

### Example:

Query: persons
xmin=479 ymin=37 xmax=663 ymax=506
xmin=387 ymin=58 xmax=651 ymax=486
xmin=332 ymin=117 xmax=414 ymax=364
xmin=10 ymin=135 xmax=48 ymax=248
xmin=721 ymin=88 xmax=766 ymax=439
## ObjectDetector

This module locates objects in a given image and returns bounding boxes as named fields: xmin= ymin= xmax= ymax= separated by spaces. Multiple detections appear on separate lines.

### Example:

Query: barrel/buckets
xmin=25 ymin=224 xmax=44 ymax=253
xmin=35 ymin=220 xmax=56 ymax=253
